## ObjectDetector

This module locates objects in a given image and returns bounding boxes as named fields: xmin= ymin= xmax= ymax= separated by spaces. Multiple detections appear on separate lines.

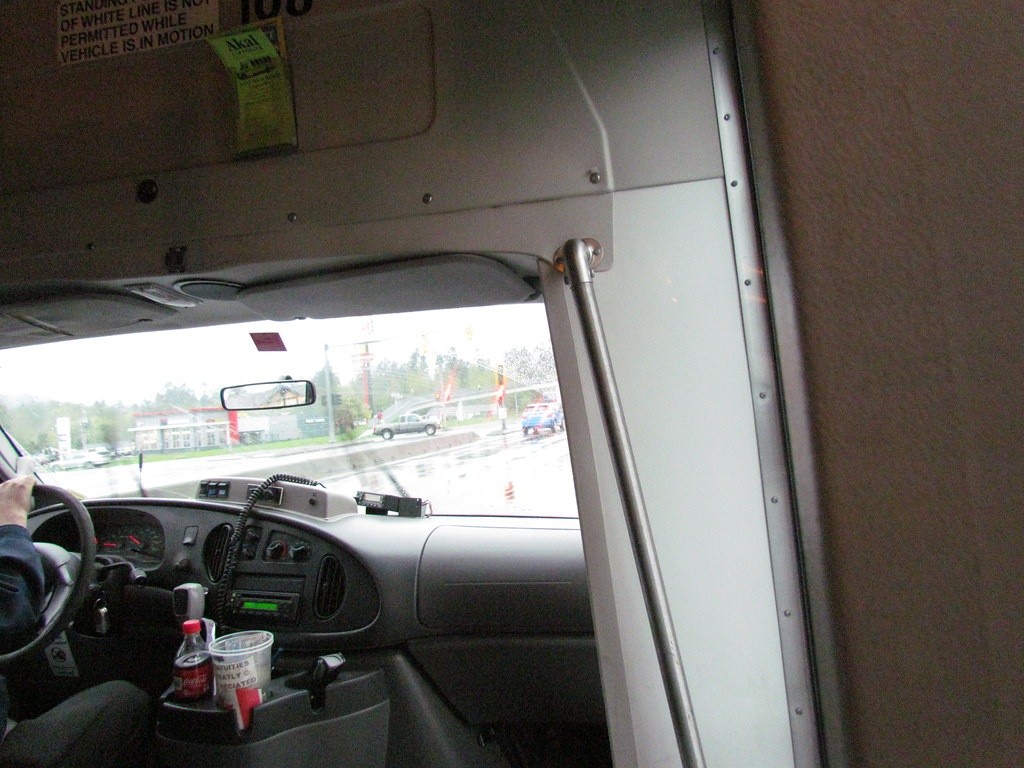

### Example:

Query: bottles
xmin=173 ymin=620 xmax=215 ymax=703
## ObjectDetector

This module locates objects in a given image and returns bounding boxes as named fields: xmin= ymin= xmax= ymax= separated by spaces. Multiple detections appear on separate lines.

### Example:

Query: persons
xmin=0 ymin=476 xmax=155 ymax=768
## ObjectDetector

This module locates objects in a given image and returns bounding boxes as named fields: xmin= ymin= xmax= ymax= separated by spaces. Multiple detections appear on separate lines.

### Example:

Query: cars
xmin=522 ymin=404 xmax=565 ymax=435
xmin=373 ymin=413 xmax=441 ymax=440
xmin=34 ymin=445 xmax=131 ymax=471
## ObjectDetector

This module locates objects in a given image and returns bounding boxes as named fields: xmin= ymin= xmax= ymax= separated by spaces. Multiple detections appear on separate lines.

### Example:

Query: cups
xmin=207 ymin=629 xmax=274 ymax=733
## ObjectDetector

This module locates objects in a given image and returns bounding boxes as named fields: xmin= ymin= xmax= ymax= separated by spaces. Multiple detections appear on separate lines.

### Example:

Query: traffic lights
xmin=332 ymin=393 xmax=342 ymax=406
